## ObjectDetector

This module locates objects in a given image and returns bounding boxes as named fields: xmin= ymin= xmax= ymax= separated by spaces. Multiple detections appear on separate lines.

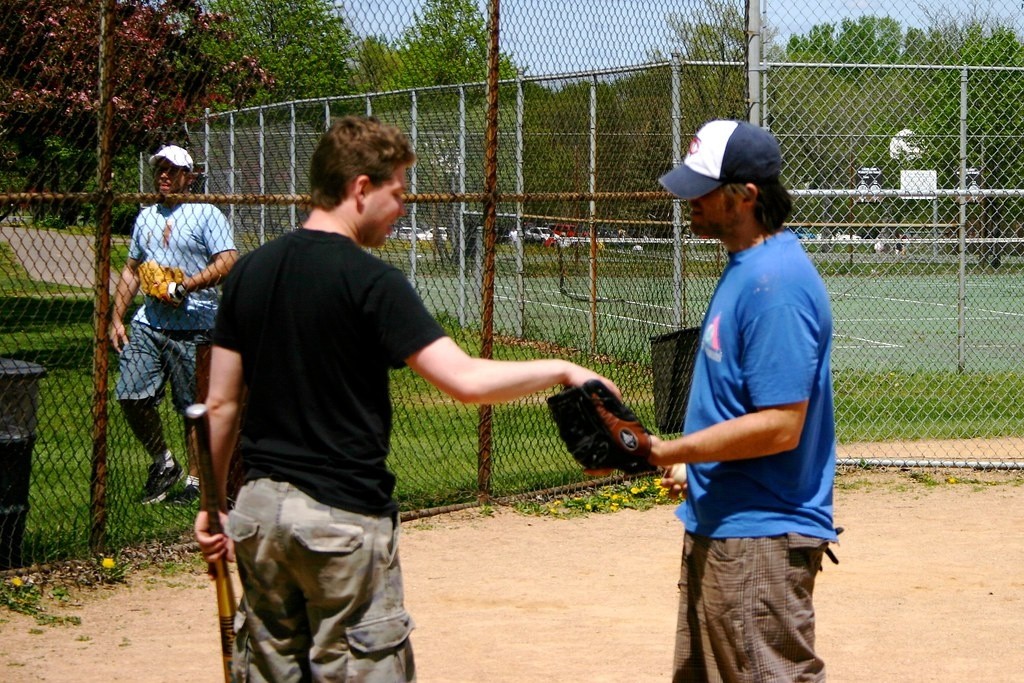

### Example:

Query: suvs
xmin=542 ymin=223 xmax=577 ymax=236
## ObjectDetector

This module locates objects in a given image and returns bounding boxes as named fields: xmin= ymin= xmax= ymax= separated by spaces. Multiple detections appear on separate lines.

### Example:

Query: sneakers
xmin=140 ymin=454 xmax=185 ymax=504
xmin=165 ymin=484 xmax=200 ymax=509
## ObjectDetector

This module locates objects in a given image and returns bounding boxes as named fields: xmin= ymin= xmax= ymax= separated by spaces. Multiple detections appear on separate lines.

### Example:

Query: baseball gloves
xmin=138 ymin=261 xmax=187 ymax=308
xmin=544 ymin=381 xmax=661 ymax=478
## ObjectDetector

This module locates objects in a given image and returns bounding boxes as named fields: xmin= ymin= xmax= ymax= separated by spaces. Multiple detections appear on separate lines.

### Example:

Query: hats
xmin=659 ymin=120 xmax=781 ymax=199
xmin=148 ymin=145 xmax=193 ymax=173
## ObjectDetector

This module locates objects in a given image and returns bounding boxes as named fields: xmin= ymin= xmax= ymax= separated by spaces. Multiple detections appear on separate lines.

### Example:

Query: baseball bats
xmin=187 ymin=404 xmax=239 ymax=683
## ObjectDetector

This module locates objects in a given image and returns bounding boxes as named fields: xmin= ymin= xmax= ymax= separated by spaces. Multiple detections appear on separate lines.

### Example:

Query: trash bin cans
xmin=0 ymin=357 xmax=48 ymax=570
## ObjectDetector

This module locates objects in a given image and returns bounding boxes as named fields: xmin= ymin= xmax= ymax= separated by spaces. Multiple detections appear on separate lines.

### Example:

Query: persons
xmin=193 ymin=117 xmax=627 ymax=682
xmin=106 ymin=144 xmax=240 ymax=507
xmin=643 ymin=118 xmax=844 ymax=682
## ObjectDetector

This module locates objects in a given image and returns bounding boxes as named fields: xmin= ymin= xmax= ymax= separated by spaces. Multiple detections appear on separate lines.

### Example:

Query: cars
xmin=525 ymin=227 xmax=560 ymax=245
xmin=386 ymin=221 xmax=448 ymax=240
